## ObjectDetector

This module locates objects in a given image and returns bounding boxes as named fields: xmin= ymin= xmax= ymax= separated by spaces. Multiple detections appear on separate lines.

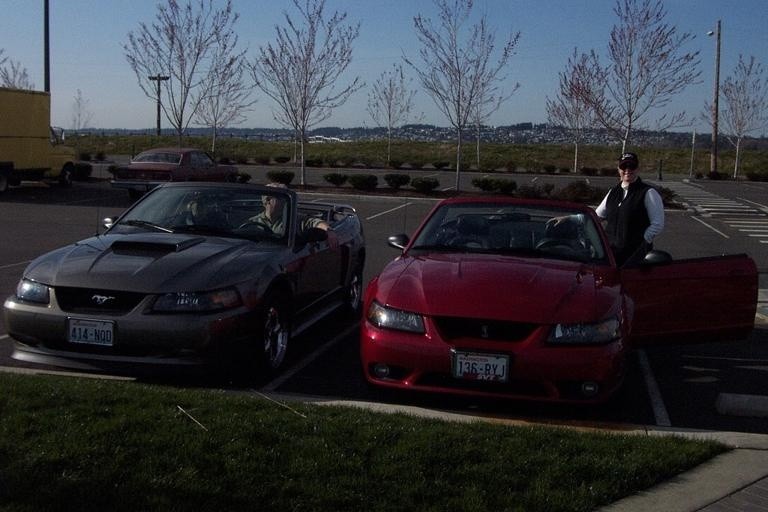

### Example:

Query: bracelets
xmin=325 ymin=228 xmax=334 ymax=233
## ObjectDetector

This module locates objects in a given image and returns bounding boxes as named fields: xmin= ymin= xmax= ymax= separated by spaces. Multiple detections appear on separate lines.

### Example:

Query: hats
xmin=618 ymin=152 xmax=638 ymax=166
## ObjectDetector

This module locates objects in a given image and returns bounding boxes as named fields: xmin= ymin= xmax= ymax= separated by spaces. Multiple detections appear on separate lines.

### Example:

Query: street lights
xmin=705 ymin=20 xmax=722 ymax=174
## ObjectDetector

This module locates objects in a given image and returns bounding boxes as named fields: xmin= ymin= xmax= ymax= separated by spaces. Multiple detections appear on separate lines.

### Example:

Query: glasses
xmin=619 ymin=163 xmax=638 ymax=170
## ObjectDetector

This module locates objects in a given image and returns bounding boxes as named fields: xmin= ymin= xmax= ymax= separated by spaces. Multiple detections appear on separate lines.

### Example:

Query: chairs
xmin=446 ymin=214 xmax=584 ymax=252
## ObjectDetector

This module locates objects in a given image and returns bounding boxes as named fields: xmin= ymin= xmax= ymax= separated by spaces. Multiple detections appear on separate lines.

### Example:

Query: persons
xmin=171 ymin=191 xmax=228 ymax=231
xmin=544 ymin=152 xmax=666 ymax=261
xmin=247 ymin=182 xmax=340 ymax=254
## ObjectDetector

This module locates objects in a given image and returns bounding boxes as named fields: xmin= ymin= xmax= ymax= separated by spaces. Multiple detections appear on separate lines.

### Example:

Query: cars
xmin=359 ymin=193 xmax=760 ymax=408
xmin=107 ymin=146 xmax=239 ymax=185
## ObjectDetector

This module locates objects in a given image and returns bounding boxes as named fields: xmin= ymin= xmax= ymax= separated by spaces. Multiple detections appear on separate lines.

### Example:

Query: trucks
xmin=0 ymin=86 xmax=77 ymax=193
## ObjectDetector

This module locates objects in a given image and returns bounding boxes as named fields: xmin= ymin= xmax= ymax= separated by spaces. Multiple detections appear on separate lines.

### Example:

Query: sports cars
xmin=3 ymin=179 xmax=366 ymax=381
